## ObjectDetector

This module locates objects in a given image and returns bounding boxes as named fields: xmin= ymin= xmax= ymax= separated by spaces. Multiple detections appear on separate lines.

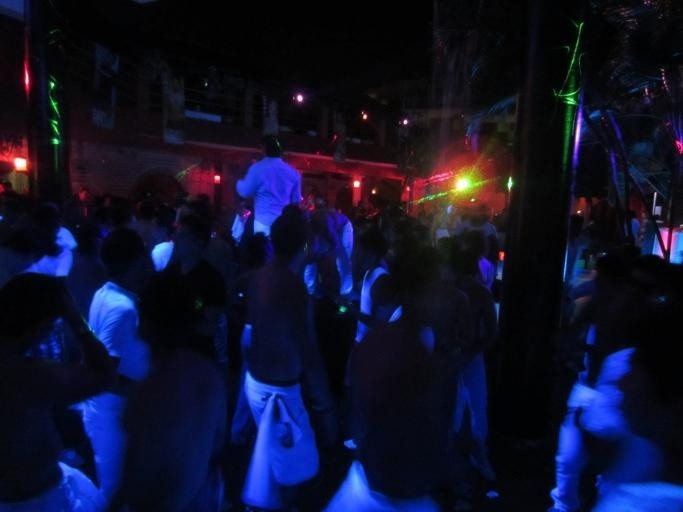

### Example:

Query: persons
xmin=234 ymin=136 xmax=303 ymax=244
xmin=1 ymin=190 xmax=498 ymax=511
xmin=552 ymin=209 xmax=683 ymax=512
